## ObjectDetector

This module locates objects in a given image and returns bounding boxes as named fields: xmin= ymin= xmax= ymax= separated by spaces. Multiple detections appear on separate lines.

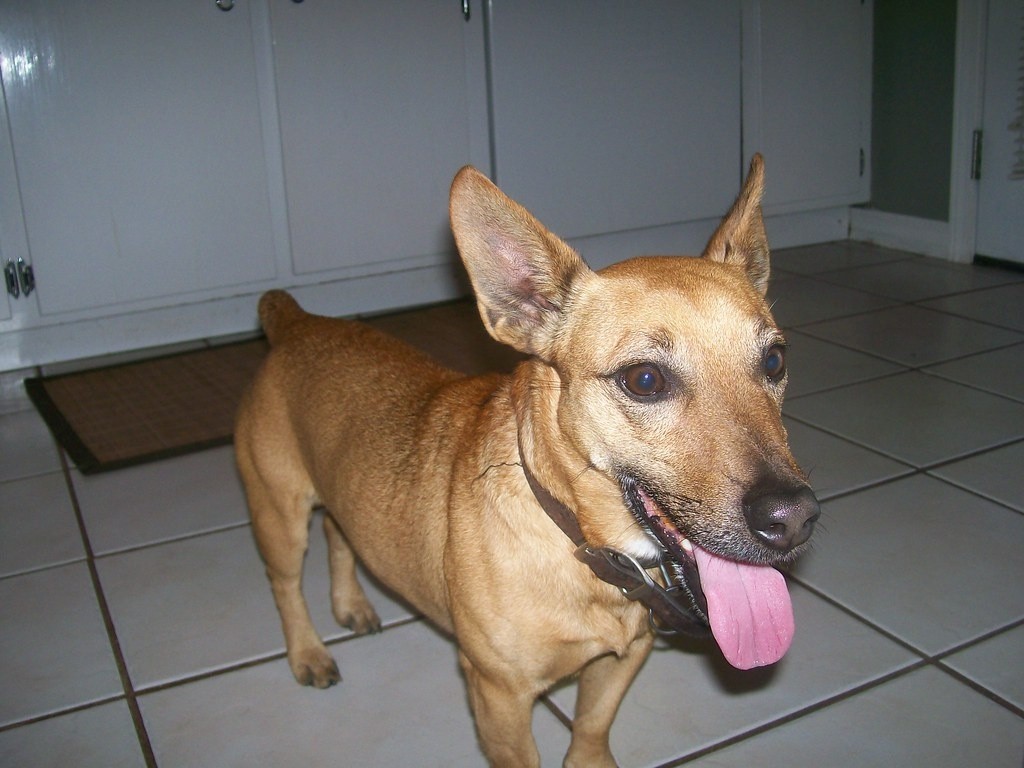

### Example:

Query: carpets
xmin=25 ymin=294 xmax=534 ymax=473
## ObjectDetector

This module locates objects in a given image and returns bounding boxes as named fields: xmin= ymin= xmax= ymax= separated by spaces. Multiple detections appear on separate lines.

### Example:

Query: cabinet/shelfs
xmin=0 ymin=1 xmax=872 ymax=372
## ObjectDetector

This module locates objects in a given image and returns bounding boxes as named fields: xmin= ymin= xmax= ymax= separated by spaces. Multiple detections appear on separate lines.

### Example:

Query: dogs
xmin=233 ymin=149 xmax=821 ymax=768
